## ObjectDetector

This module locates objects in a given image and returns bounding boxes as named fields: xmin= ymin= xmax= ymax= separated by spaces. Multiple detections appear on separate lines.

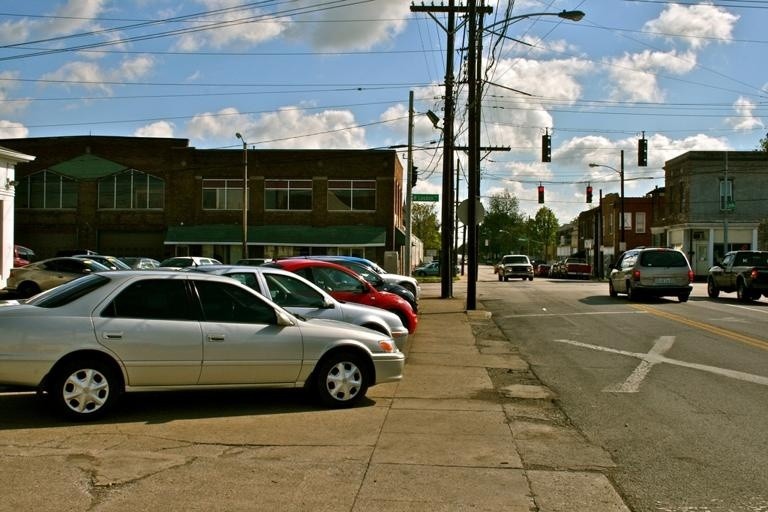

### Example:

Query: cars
xmin=233 ymin=259 xmax=273 ymax=269
xmin=317 ymin=256 xmax=420 ymax=301
xmin=303 ymin=257 xmax=417 ymax=314
xmin=73 ymin=254 xmax=132 ymax=271
xmin=259 ymin=258 xmax=418 ymax=334
xmin=0 ymin=271 xmax=405 ymax=415
xmin=154 ymin=256 xmax=224 ymax=271
xmin=175 ymin=257 xmax=409 ymax=352
xmin=413 ymin=262 xmax=460 ymax=277
xmin=6 ymin=257 xmax=113 ymax=299
xmin=117 ymin=257 xmax=162 ymax=269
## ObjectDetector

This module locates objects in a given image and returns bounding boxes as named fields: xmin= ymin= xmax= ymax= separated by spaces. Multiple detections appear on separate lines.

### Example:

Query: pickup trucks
xmin=494 ymin=255 xmax=535 ymax=282
xmin=706 ymin=250 xmax=768 ymax=304
xmin=548 ymin=258 xmax=592 ymax=278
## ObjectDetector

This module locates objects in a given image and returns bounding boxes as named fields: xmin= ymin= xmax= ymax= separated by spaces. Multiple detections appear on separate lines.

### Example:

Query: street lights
xmin=477 ymin=10 xmax=584 ymax=279
xmin=466 ymin=8 xmax=586 ymax=311
xmin=236 ymin=132 xmax=249 ymax=258
xmin=235 ymin=131 xmax=247 ymax=255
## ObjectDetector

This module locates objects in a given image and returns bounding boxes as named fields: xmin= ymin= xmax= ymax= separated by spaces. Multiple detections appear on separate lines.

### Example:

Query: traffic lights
xmin=541 ymin=135 xmax=552 ymax=162
xmin=586 ymin=185 xmax=592 ymax=202
xmin=587 ymin=187 xmax=592 ymax=202
xmin=542 ymin=135 xmax=551 ymax=162
xmin=538 ymin=186 xmax=545 ymax=203
xmin=537 ymin=186 xmax=545 ymax=203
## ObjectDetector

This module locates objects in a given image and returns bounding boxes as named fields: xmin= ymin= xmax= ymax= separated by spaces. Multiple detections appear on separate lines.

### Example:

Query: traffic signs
xmin=725 ymin=200 xmax=735 ymax=210
xmin=412 ymin=194 xmax=439 ymax=201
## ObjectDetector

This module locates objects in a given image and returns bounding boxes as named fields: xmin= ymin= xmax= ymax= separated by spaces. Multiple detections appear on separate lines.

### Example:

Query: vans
xmin=608 ymin=248 xmax=693 ymax=302
xmin=14 ymin=244 xmax=35 ymax=266
